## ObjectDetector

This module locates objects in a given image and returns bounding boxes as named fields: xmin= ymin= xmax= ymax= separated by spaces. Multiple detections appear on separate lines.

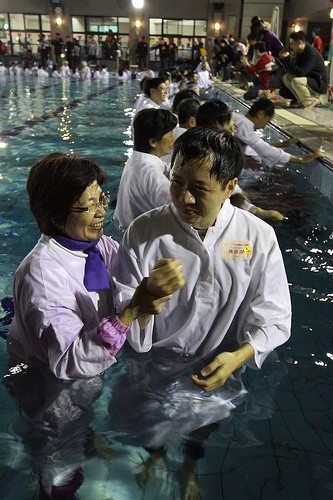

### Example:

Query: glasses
xmin=68 ymin=191 xmax=110 ymax=212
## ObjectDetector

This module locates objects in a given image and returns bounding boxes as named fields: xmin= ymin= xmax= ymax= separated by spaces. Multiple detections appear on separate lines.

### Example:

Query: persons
xmin=106 ymin=126 xmax=292 ymax=500
xmin=111 ymin=108 xmax=178 ymax=231
xmin=0 ymin=16 xmax=333 ymax=169
xmin=196 ymin=98 xmax=285 ymax=222
xmin=6 ymin=152 xmax=172 ymax=460
